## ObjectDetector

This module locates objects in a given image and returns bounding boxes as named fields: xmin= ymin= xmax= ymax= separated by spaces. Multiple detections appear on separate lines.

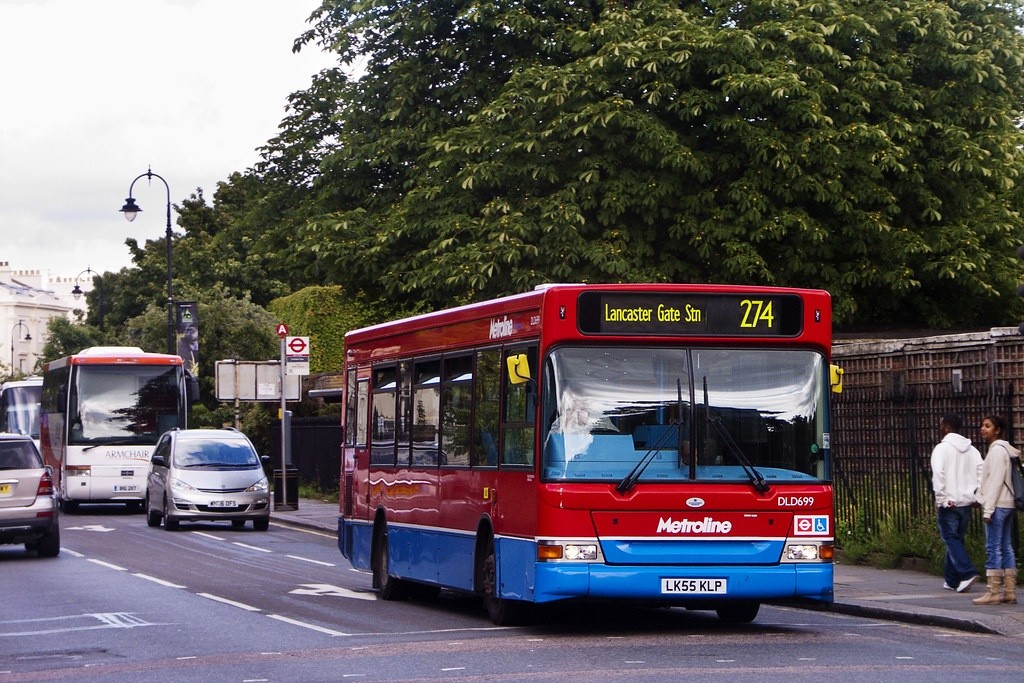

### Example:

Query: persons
xmin=929 ymin=413 xmax=984 ymax=592
xmin=974 ymin=415 xmax=1021 ymax=606
xmin=543 ymin=375 xmax=621 ymax=454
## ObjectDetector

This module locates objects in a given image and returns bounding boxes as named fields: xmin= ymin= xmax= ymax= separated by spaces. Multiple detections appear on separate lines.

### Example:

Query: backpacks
xmin=993 ymin=444 xmax=1024 ymax=510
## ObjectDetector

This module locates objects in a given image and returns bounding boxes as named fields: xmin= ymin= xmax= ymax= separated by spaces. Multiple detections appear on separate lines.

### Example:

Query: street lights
xmin=118 ymin=171 xmax=177 ymax=356
xmin=72 ymin=269 xmax=105 ymax=332
xmin=10 ymin=322 xmax=32 ymax=381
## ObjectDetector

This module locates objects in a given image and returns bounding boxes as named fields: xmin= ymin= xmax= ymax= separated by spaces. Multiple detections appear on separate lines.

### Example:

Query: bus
xmin=0 ymin=376 xmax=45 ymax=456
xmin=37 ymin=345 xmax=203 ymax=513
xmin=334 ymin=283 xmax=848 ymax=629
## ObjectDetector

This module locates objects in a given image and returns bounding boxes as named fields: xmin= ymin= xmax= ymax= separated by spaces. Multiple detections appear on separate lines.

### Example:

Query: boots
xmin=999 ymin=569 xmax=1018 ymax=604
xmin=971 ymin=570 xmax=1004 ymax=606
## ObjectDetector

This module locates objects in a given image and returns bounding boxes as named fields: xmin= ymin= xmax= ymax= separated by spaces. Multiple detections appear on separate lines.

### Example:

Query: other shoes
xmin=956 ymin=575 xmax=979 ymax=592
xmin=943 ymin=582 xmax=956 ymax=590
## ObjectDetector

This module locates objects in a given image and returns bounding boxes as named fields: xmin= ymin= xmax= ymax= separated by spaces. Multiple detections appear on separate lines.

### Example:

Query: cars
xmin=0 ymin=427 xmax=61 ymax=557
xmin=146 ymin=427 xmax=275 ymax=532
